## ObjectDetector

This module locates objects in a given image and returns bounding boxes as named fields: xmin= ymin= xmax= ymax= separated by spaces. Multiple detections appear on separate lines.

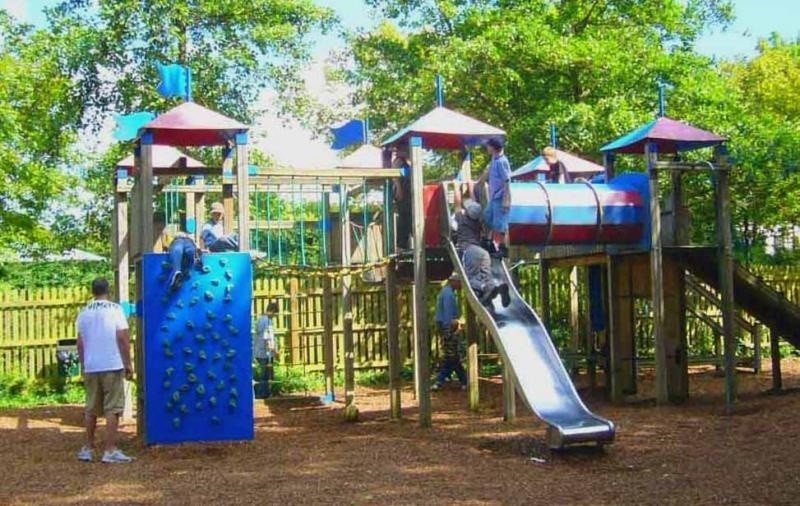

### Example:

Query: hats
xmin=466 ymin=201 xmax=482 ymax=221
xmin=482 ymin=136 xmax=504 ymax=148
xmin=209 ymin=203 xmax=224 ymax=214
xmin=448 ymin=273 xmax=461 ymax=281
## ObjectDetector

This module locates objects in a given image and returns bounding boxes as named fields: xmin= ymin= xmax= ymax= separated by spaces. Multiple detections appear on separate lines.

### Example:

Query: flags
xmin=155 ymin=57 xmax=189 ymax=101
xmin=110 ymin=112 xmax=158 ymax=141
xmin=329 ymin=119 xmax=367 ymax=150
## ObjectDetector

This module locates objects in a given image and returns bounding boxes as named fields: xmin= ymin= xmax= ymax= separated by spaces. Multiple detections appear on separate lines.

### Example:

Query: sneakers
xmin=494 ymin=247 xmax=508 ymax=258
xmin=101 ymin=449 xmax=134 ymax=464
xmin=76 ymin=445 xmax=97 ymax=462
xmin=482 ymin=286 xmax=498 ymax=306
xmin=498 ymin=284 xmax=511 ymax=307
xmin=168 ymin=271 xmax=184 ymax=291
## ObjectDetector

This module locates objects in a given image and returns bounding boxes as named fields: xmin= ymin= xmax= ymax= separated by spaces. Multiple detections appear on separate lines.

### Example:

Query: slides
xmin=442 ymin=235 xmax=616 ymax=449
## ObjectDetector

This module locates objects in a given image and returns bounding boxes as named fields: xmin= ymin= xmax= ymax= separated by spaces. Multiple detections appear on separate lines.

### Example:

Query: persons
xmin=162 ymin=223 xmax=196 ymax=288
xmin=198 ymin=200 xmax=268 ymax=261
xmin=430 ymin=273 xmax=470 ymax=392
xmin=390 ymin=155 xmax=414 ymax=250
xmin=449 ymin=178 xmax=512 ymax=308
xmin=74 ymin=277 xmax=138 ymax=463
xmin=479 ymin=137 xmax=512 ymax=258
xmin=254 ymin=300 xmax=285 ymax=398
xmin=543 ymin=146 xmax=573 ymax=183
